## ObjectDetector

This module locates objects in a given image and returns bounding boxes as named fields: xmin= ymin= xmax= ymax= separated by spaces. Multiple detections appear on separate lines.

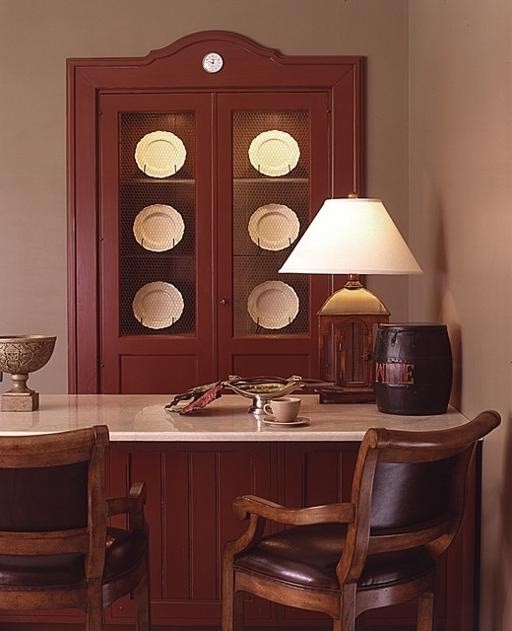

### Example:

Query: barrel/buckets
xmin=372 ymin=321 xmax=453 ymax=415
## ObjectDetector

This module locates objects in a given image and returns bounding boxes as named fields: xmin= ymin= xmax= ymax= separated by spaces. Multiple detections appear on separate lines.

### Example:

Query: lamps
xmin=279 ymin=191 xmax=426 ymax=407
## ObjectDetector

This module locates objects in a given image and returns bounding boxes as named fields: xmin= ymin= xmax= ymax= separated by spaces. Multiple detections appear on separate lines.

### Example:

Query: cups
xmin=264 ymin=399 xmax=301 ymax=419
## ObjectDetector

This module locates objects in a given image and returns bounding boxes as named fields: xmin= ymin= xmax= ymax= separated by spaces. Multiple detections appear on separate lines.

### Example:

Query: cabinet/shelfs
xmin=63 ymin=27 xmax=372 ymax=628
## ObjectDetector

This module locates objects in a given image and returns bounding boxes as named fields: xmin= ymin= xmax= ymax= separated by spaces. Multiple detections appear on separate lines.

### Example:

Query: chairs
xmin=223 ymin=407 xmax=502 ymax=629
xmin=1 ymin=421 xmax=152 ymax=629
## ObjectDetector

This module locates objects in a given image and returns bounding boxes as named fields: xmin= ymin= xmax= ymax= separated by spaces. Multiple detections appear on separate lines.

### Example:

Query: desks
xmin=1 ymin=389 xmax=488 ymax=631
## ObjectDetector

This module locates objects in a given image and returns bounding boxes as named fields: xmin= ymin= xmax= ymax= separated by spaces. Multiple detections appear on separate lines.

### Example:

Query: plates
xmin=247 ymin=281 xmax=299 ymax=329
xmin=247 ymin=205 xmax=299 ymax=251
xmin=134 ymin=132 xmax=188 ymax=177
xmin=248 ymin=130 xmax=301 ymax=177
xmin=132 ymin=205 xmax=184 ymax=250
xmin=132 ymin=282 xmax=184 ymax=330
xmin=260 ymin=418 xmax=310 ymax=430
